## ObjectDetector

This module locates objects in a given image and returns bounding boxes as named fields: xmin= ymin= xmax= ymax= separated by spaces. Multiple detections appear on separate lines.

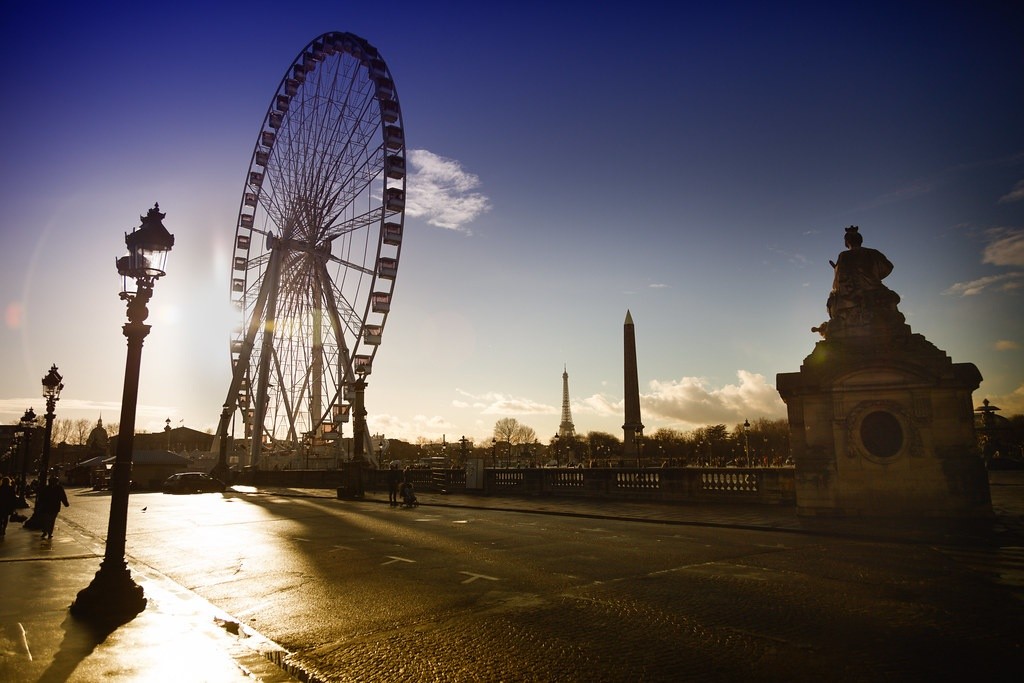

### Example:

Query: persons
xmin=826 ymin=225 xmax=899 ymax=316
xmin=386 ymin=465 xmax=399 ymax=506
xmin=0 ymin=477 xmax=18 ymax=537
xmin=696 ymin=455 xmax=783 ymax=467
xmin=39 ymin=477 xmax=69 ymax=539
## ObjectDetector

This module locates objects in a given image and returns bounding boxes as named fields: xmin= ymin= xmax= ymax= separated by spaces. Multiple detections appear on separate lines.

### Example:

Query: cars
xmin=162 ymin=472 xmax=227 ymax=495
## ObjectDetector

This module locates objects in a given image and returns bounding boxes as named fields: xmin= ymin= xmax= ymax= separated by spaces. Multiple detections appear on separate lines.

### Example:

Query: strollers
xmin=399 ymin=483 xmax=419 ymax=508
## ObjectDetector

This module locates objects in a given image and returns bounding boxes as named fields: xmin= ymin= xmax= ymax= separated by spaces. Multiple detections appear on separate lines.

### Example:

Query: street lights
xmin=305 ymin=440 xmax=312 ymax=469
xmin=492 ymin=438 xmax=497 ymax=469
xmin=22 ymin=362 xmax=64 ymax=530
xmin=442 ymin=442 xmax=447 ymax=468
xmin=634 ymin=425 xmax=641 ymax=467
xmin=67 ymin=202 xmax=175 ymax=619
xmin=378 ymin=440 xmax=383 ymax=471
xmin=0 ymin=407 xmax=37 ymax=508
xmin=555 ymin=432 xmax=560 ymax=468
xmin=744 ymin=418 xmax=750 ymax=467
xmin=164 ymin=417 xmax=171 ymax=451
